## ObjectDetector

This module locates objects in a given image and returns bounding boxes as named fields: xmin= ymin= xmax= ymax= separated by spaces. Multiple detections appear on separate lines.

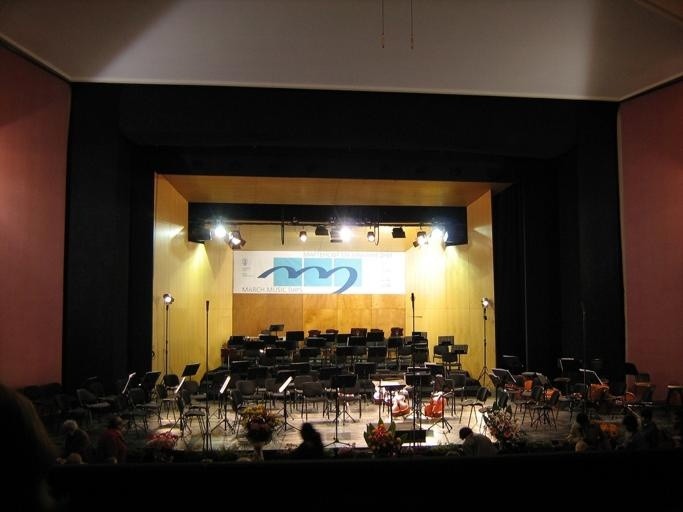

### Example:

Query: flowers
xmin=483 ymin=402 xmax=521 ymax=450
xmin=143 ymin=433 xmax=178 ymax=465
xmin=241 ymin=400 xmax=283 ymax=461
xmin=364 ymin=416 xmax=408 ymax=459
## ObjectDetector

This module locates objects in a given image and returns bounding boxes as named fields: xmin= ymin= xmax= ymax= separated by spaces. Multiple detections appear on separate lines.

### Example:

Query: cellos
xmin=425 ymin=391 xmax=446 ymax=418
xmin=391 ymin=392 xmax=409 ymax=416
xmin=373 ymin=380 xmax=387 ymax=404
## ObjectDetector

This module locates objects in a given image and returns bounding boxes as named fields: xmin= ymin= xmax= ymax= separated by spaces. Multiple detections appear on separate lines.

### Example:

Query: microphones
xmin=580 ymin=300 xmax=587 ymax=313
xmin=206 ymin=300 xmax=209 ymax=311
xmin=411 ymin=293 xmax=414 ymax=301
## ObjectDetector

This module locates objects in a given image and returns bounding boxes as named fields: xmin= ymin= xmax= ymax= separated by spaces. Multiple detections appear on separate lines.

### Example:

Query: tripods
xmin=478 ymin=309 xmax=491 ymax=387
xmin=116 ymin=388 xmax=626 ymax=452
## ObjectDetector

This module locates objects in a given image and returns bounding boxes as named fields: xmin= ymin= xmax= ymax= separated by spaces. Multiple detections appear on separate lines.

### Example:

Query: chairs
xmin=23 ymin=331 xmax=600 ymax=432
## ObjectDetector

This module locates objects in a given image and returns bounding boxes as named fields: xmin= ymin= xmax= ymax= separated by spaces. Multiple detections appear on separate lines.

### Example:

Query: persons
xmin=567 ymin=408 xmax=681 ymax=451
xmin=54 ymin=415 xmax=128 ymax=465
xmin=459 ymin=427 xmax=498 ymax=456
xmin=287 ymin=421 xmax=323 ymax=459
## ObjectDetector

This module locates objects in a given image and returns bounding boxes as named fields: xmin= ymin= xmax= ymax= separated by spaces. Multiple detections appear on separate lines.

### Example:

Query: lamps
xmin=481 ymin=297 xmax=490 ymax=309
xmin=163 ymin=293 xmax=175 ymax=305
xmin=367 ymin=223 xmax=375 ymax=242
xmin=413 ymin=224 xmax=428 ymax=248
xmin=299 ymin=223 xmax=307 ymax=241
xmin=224 ymin=222 xmax=246 ymax=250
